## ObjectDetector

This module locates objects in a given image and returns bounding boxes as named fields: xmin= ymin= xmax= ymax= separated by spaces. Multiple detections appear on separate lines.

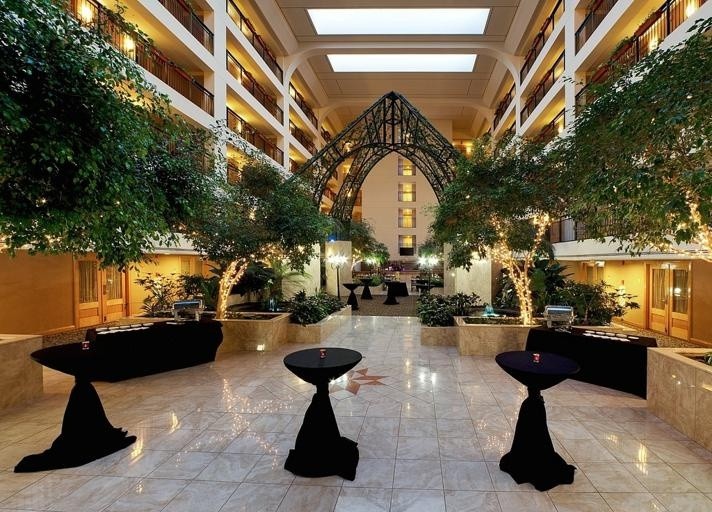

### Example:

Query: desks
xmin=283 ymin=346 xmax=362 ymax=485
xmin=343 ymin=278 xmax=436 ymax=310
xmin=85 ymin=317 xmax=224 ymax=383
xmin=524 ymin=324 xmax=658 ymax=400
xmin=11 ymin=342 xmax=137 ymax=474
xmin=493 ymin=350 xmax=581 ymax=490
